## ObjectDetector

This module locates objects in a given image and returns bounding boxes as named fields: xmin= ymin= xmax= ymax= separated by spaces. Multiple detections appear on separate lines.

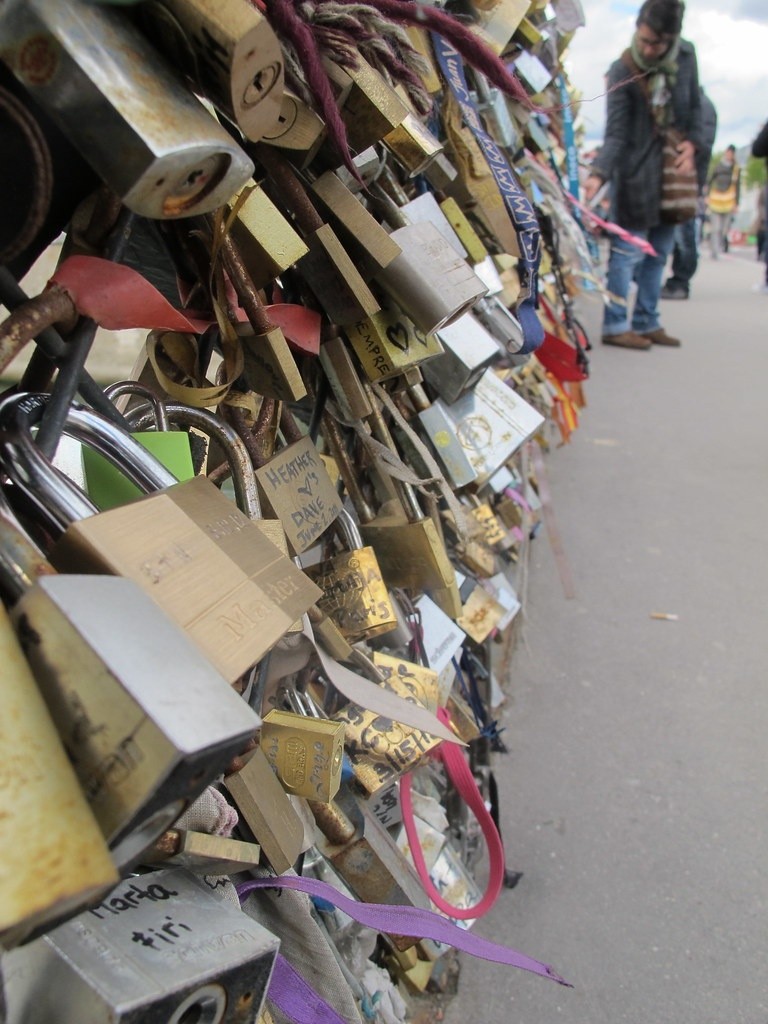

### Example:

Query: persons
xmin=706 ymin=145 xmax=741 ymax=254
xmin=581 ymin=148 xmax=611 ymax=265
xmin=752 ymin=121 xmax=768 ymax=294
xmin=582 ymin=0 xmax=703 ymax=348
xmin=660 ymin=85 xmax=716 ymax=300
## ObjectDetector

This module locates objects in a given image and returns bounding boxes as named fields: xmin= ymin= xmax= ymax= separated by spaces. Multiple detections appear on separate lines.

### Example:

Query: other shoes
xmin=661 ymin=287 xmax=689 ymax=299
xmin=601 ymin=331 xmax=651 ymax=350
xmin=640 ymin=328 xmax=681 ymax=347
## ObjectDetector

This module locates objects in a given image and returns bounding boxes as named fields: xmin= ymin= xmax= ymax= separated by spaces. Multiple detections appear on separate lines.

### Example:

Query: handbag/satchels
xmin=707 ymin=187 xmax=737 ymax=214
